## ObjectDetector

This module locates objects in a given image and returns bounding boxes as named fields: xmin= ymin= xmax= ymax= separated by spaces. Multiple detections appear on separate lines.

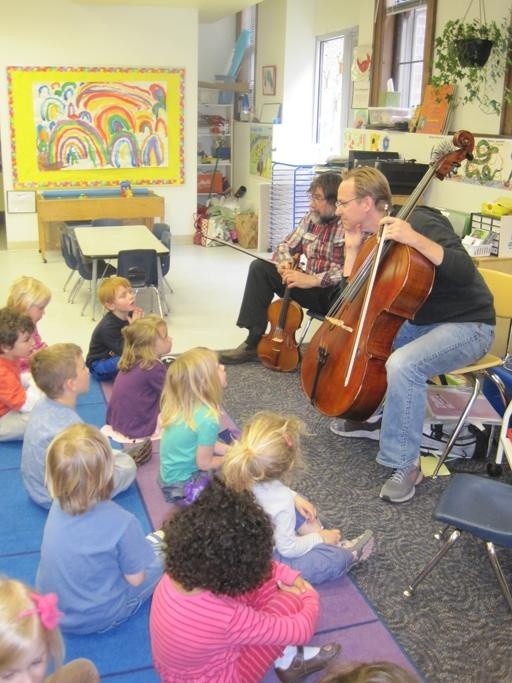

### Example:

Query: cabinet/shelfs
xmin=265 ymin=158 xmax=325 ymax=252
xmin=388 ymin=193 xmax=429 ymax=213
xmin=197 ymin=100 xmax=235 ymax=211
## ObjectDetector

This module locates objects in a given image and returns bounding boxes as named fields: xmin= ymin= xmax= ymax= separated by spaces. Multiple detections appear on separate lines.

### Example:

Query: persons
xmin=0 ymin=308 xmax=46 ymax=443
xmin=157 ymin=346 xmax=233 ymax=511
xmin=329 ymin=167 xmax=497 ymax=505
xmin=0 ymin=578 xmax=101 ymax=683
xmin=323 ymin=659 xmax=423 ymax=683
xmin=85 ymin=278 xmax=145 ymax=381
xmin=19 ymin=342 xmax=153 ymax=513
xmin=219 ymin=172 xmax=344 ymax=364
xmin=104 ymin=312 xmax=173 ymax=466
xmin=150 ymin=487 xmax=342 ymax=683
xmin=0 ymin=275 xmax=52 ymax=374
xmin=218 ymin=410 xmax=376 ymax=587
xmin=34 ymin=422 xmax=167 ymax=636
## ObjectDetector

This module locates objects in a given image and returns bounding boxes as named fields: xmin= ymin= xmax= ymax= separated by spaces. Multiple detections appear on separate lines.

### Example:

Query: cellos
xmin=299 ymin=130 xmax=476 ymax=419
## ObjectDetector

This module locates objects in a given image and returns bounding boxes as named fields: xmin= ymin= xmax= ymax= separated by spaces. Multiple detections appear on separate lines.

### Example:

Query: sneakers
xmin=329 ymin=416 xmax=382 ymax=441
xmin=119 ymin=438 xmax=152 ymax=467
xmin=334 ymin=528 xmax=375 ymax=574
xmin=379 ymin=457 xmax=424 ymax=504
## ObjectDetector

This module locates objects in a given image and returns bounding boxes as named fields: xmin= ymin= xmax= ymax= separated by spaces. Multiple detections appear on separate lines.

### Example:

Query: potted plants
xmin=430 ymin=13 xmax=512 ymax=118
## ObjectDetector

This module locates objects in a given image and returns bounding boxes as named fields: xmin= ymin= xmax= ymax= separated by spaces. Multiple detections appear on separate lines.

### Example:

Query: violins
xmin=256 ymin=253 xmax=303 ymax=373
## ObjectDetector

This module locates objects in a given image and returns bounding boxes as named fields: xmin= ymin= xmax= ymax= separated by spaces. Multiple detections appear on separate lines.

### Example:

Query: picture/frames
xmin=4 ymin=188 xmax=37 ymax=215
xmin=261 ymin=63 xmax=276 ymax=96
xmin=258 ymin=102 xmax=281 ymax=124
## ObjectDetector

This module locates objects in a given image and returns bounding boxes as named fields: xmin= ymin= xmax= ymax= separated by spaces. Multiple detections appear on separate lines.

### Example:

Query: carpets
xmin=215 ymin=352 xmax=512 ymax=683
xmin=1 ymin=348 xmax=426 ymax=682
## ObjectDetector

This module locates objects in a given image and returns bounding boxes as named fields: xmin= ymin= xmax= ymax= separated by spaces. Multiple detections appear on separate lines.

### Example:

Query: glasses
xmin=334 ymin=197 xmax=359 ymax=210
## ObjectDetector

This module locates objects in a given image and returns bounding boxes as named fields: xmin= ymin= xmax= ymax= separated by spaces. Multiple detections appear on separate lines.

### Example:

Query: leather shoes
xmin=273 ymin=643 xmax=342 ymax=683
xmin=218 ymin=341 xmax=261 ymax=365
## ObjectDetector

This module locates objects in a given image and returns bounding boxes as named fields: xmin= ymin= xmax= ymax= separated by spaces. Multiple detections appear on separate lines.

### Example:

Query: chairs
xmin=400 ymin=468 xmax=511 ymax=612
xmin=417 ymin=261 xmax=512 ymax=490
xmin=55 ymin=212 xmax=176 ymax=322
xmin=298 ymin=305 xmax=328 ymax=351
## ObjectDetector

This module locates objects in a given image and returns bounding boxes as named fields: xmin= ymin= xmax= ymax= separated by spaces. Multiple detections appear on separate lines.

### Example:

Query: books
xmin=201 ymin=158 xmax=231 ymax=164
xmin=415 ymin=84 xmax=459 ymax=136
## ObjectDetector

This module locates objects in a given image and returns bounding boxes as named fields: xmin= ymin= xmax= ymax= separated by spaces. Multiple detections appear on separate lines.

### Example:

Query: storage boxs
xmin=366 ymin=107 xmax=412 ymax=128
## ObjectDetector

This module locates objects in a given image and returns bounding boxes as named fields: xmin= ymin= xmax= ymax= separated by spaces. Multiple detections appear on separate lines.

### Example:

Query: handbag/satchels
xmin=193 ymin=213 xmax=233 ymax=247
xmin=235 ymin=208 xmax=258 ymax=249
xmin=419 ymin=422 xmax=477 ymax=463
